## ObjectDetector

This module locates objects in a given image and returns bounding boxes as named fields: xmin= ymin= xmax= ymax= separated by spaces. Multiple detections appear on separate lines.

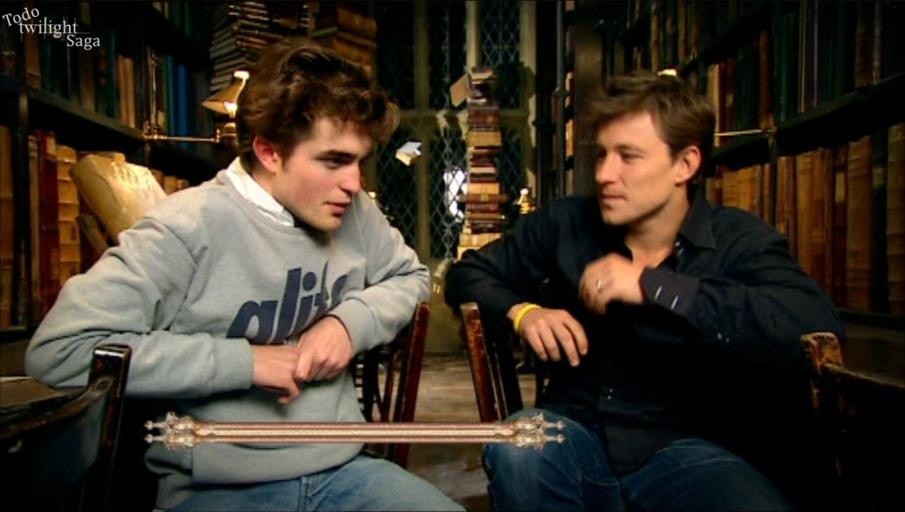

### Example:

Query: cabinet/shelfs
xmin=580 ymin=0 xmax=905 ymax=381
xmin=0 ymin=0 xmax=224 ymax=335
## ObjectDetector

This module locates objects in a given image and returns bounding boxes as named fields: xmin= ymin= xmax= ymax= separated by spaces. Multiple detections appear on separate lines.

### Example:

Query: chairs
xmin=796 ymin=325 xmax=905 ymax=512
xmin=347 ymin=300 xmax=431 ymax=473
xmin=0 ymin=336 xmax=135 ymax=511
xmin=455 ymin=295 xmax=553 ymax=445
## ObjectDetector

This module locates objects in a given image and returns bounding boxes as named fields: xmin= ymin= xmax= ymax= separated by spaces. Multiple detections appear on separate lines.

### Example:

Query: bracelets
xmin=513 ymin=303 xmax=543 ymax=337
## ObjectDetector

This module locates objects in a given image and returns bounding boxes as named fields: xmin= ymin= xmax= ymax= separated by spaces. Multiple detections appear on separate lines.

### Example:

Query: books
xmin=208 ymin=1 xmax=386 ymax=167
xmin=600 ymin=1 xmax=903 ymax=322
xmin=458 ymin=65 xmax=505 ymax=262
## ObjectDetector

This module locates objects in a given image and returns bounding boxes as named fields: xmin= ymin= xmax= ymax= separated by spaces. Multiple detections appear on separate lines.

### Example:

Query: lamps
xmin=135 ymin=67 xmax=250 ymax=148
xmin=512 ymin=185 xmax=539 ymax=215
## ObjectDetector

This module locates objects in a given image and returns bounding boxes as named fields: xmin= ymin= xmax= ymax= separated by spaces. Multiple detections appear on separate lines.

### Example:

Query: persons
xmin=1 ymin=1 xmax=204 ymax=328
xmin=24 ymin=37 xmax=473 ymax=511
xmin=441 ymin=66 xmax=837 ymax=509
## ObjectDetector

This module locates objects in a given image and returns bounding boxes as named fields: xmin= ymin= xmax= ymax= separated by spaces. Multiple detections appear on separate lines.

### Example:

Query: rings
xmin=596 ymin=280 xmax=605 ymax=289
